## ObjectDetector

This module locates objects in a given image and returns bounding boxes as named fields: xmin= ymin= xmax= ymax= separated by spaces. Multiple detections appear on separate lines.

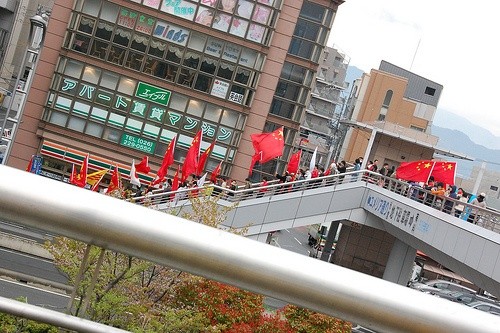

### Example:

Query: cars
xmin=407 ymin=261 xmax=500 ymax=316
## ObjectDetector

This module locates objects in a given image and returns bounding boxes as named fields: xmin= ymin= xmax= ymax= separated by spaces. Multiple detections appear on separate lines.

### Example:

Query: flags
xmin=155 ymin=138 xmax=175 ymax=184
xmin=430 ymin=162 xmax=455 ymax=185
xmin=287 ymin=147 xmax=317 ymax=173
xmin=130 ymin=160 xmax=141 ymax=187
xmin=170 ymin=167 xmax=179 ymax=200
xmin=136 ymin=158 xmax=152 ymax=174
xmin=196 ymin=141 xmax=216 ymax=176
xmin=197 ymin=173 xmax=208 ymax=187
xmin=249 ymin=127 xmax=284 ymax=175
xmin=71 ymin=154 xmax=118 ymax=193
xmin=181 ymin=129 xmax=202 ymax=183
xmin=211 ymin=161 xmax=223 ymax=184
xmin=395 ymin=161 xmax=436 ymax=182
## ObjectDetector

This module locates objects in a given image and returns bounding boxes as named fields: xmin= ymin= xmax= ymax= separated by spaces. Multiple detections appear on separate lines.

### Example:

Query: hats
xmin=480 ymin=193 xmax=486 ymax=197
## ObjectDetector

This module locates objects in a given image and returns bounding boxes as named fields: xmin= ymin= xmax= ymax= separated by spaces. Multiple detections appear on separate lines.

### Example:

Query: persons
xmin=196 ymin=0 xmax=239 ymax=32
xmin=275 ymin=157 xmax=363 ymax=195
xmin=154 ymin=175 xmax=201 ymax=203
xmin=215 ymin=176 xmax=237 ymax=201
xmin=367 ymin=159 xmax=486 ymax=222
xmin=256 ymin=177 xmax=268 ymax=198
xmin=239 ymin=178 xmax=251 ymax=201
